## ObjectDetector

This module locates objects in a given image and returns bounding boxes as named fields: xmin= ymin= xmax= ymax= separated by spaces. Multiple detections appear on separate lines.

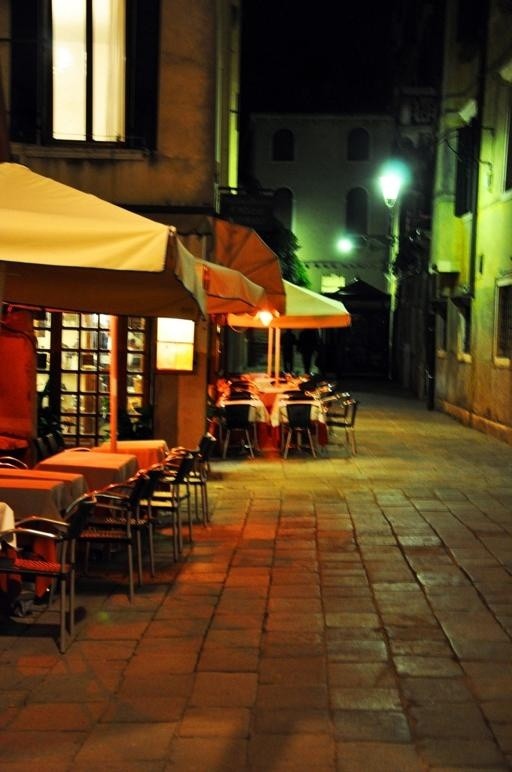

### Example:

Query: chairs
xmin=0 ymin=440 xmax=90 ymax=469
xmin=75 ymin=441 xmax=207 ymax=600
xmin=207 ymin=371 xmax=359 ymax=458
xmin=0 ymin=494 xmax=96 ymax=651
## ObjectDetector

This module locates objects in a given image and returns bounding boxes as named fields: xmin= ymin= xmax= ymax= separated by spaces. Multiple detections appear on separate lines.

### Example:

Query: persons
xmin=296 ymin=329 xmax=320 ymax=375
xmin=281 ymin=329 xmax=297 ymax=372
